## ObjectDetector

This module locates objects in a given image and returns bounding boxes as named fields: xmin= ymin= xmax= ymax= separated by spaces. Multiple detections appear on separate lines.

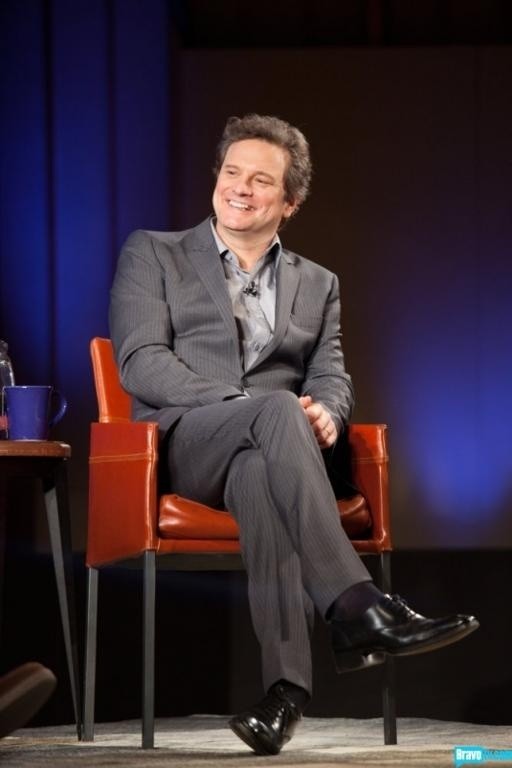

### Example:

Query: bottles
xmin=0 ymin=339 xmax=16 ymax=441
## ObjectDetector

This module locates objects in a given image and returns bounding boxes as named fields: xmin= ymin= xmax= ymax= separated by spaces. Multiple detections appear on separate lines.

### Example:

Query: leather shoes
xmin=228 ymin=685 xmax=305 ymax=754
xmin=329 ymin=593 xmax=481 ymax=675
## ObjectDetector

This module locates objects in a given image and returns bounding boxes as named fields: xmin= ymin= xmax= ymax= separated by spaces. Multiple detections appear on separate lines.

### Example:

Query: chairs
xmin=86 ymin=337 xmax=400 ymax=751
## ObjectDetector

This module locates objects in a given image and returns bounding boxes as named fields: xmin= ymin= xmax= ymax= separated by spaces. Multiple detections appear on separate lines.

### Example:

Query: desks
xmin=0 ymin=439 xmax=84 ymax=740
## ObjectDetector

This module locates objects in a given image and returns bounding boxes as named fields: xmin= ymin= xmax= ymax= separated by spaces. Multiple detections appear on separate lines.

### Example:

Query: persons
xmin=107 ymin=113 xmax=483 ymax=756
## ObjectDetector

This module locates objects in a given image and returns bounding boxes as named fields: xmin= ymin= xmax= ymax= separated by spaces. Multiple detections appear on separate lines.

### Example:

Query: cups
xmin=4 ymin=385 xmax=68 ymax=443
